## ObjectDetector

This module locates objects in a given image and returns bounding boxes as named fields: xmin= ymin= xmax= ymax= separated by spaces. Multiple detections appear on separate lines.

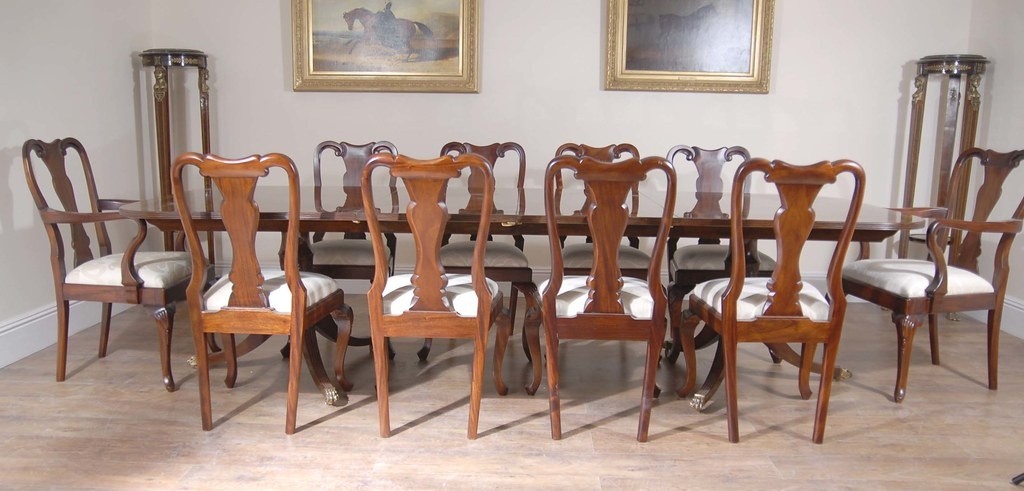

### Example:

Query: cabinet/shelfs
xmin=896 ymin=53 xmax=994 ymax=261
xmin=140 ymin=46 xmax=217 ymax=301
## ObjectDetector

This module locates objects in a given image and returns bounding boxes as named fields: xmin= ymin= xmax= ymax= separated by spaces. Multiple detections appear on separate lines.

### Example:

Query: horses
xmin=342 ymin=8 xmax=435 ymax=62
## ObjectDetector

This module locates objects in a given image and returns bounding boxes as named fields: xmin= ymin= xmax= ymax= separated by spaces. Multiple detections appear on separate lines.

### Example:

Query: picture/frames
xmin=606 ymin=1 xmax=775 ymax=94
xmin=291 ymin=0 xmax=481 ymax=92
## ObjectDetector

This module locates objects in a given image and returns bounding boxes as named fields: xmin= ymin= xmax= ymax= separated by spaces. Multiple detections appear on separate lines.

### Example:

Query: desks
xmin=121 ymin=185 xmax=924 ymax=404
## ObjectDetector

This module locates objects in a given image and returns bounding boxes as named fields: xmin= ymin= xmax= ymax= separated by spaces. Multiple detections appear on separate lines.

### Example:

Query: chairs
xmin=678 ymin=158 xmax=869 ymax=443
xmin=172 ymin=151 xmax=351 ymax=435
xmin=280 ymin=139 xmax=399 ymax=362
xmin=823 ymin=147 xmax=1023 ymax=403
xmin=365 ymin=154 xmax=503 ymax=439
xmin=663 ymin=143 xmax=777 ymax=361
xmin=408 ymin=141 xmax=537 ymax=360
xmin=547 ymin=142 xmax=653 ymax=277
xmin=532 ymin=156 xmax=675 ymax=440
xmin=23 ymin=137 xmax=219 ymax=394
